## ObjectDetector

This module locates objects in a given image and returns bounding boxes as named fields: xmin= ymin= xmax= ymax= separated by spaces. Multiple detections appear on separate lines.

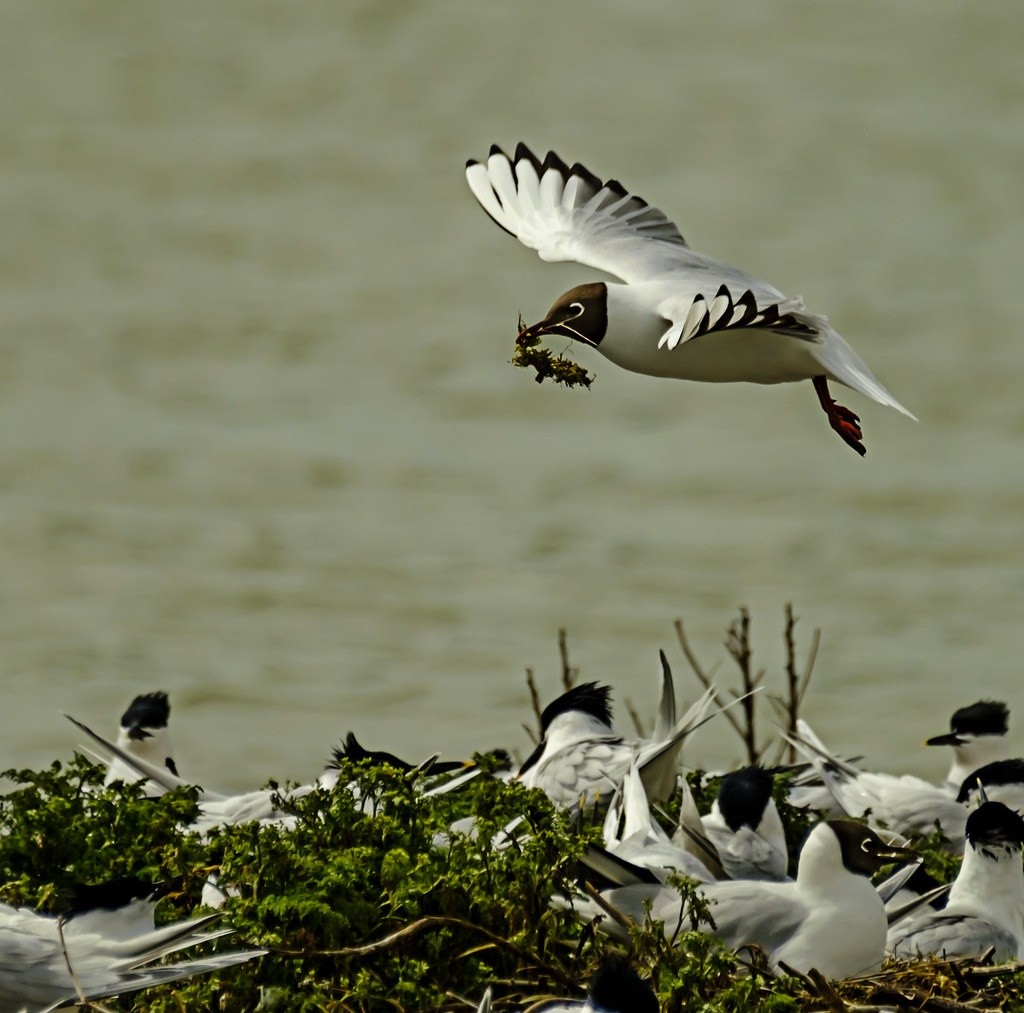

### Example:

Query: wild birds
xmin=0 ymin=678 xmax=1023 ymax=1012
xmin=465 ymin=142 xmax=921 ymax=457
